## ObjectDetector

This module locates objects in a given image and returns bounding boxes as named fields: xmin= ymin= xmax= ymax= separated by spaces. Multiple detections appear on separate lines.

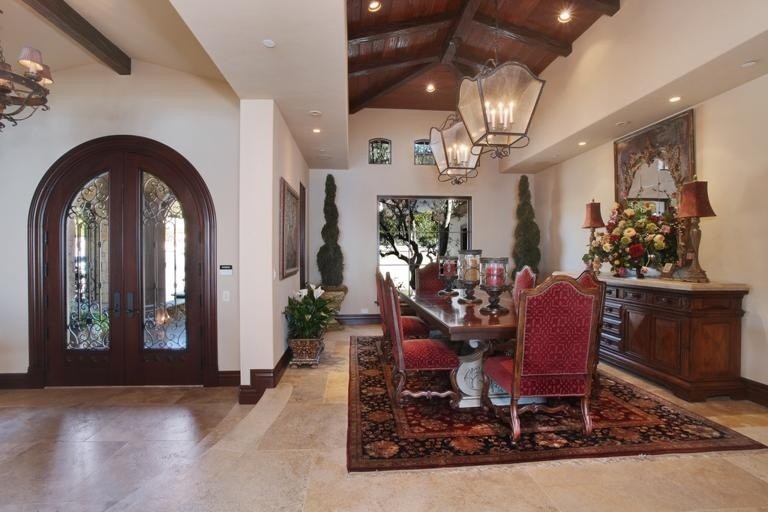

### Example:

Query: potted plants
xmin=281 ymin=282 xmax=340 ymax=369
xmin=317 ymin=173 xmax=347 ymax=331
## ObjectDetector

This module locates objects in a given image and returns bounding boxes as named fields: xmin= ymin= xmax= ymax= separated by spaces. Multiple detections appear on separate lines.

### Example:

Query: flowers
xmin=584 ymin=202 xmax=679 ymax=270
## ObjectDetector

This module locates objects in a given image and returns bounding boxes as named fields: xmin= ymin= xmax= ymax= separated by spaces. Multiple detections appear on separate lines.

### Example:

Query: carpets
xmin=346 ymin=336 xmax=767 ymax=473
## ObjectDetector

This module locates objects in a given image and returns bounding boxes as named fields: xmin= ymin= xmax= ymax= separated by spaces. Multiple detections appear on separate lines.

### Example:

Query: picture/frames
xmin=613 ymin=108 xmax=696 ymax=202
xmin=279 ymin=176 xmax=300 ymax=280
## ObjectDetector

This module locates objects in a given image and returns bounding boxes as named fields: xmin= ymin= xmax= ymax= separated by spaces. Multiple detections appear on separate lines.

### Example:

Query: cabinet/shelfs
xmin=599 ymin=284 xmax=690 ymax=402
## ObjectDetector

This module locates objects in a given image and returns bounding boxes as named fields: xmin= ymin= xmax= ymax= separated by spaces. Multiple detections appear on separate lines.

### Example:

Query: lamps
xmin=581 ymin=199 xmax=606 ymax=273
xmin=430 ymin=2 xmax=547 ymax=184
xmin=0 ymin=47 xmax=55 ymax=134
xmin=672 ymin=176 xmax=717 ymax=281
